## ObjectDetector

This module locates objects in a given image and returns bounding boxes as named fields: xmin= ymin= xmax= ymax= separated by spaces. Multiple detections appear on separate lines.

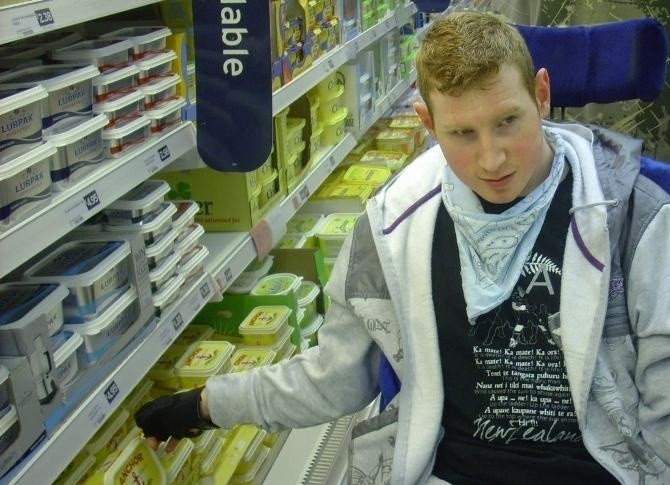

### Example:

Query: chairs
xmin=504 ymin=17 xmax=670 ymax=197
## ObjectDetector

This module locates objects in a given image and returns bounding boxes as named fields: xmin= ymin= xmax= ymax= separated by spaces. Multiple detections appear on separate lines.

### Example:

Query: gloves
xmin=134 ymin=386 xmax=220 ymax=441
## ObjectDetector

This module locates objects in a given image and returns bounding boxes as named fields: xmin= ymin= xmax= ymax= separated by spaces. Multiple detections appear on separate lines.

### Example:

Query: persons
xmin=135 ymin=9 xmax=669 ymax=484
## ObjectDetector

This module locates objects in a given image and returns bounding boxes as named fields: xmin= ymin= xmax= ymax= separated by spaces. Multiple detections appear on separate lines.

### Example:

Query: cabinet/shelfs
xmin=0 ymin=0 xmax=491 ymax=483
